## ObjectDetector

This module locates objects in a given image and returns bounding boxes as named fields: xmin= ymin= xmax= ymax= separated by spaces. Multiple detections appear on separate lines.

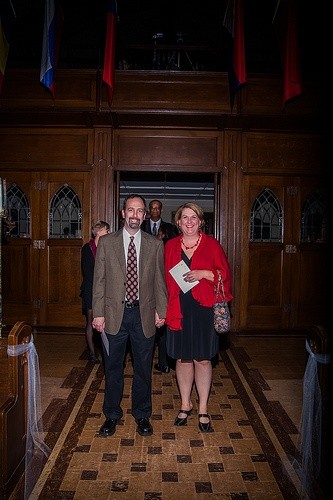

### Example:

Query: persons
xmin=79 ymin=221 xmax=111 ymax=364
xmin=154 ymin=202 xmax=233 ymax=432
xmin=139 ymin=200 xmax=178 ymax=372
xmin=92 ymin=194 xmax=168 ymax=436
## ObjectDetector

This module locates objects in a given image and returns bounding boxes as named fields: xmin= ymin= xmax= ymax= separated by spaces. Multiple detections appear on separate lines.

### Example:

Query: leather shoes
xmin=198 ymin=414 xmax=211 ymax=432
xmin=154 ymin=363 xmax=170 ymax=372
xmin=99 ymin=417 xmax=116 ymax=436
xmin=174 ymin=408 xmax=192 ymax=426
xmin=134 ymin=418 xmax=154 ymax=436
xmin=88 ymin=352 xmax=98 ymax=363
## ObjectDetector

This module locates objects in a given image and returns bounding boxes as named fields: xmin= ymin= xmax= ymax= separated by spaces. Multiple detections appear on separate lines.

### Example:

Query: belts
xmin=124 ymin=302 xmax=139 ymax=309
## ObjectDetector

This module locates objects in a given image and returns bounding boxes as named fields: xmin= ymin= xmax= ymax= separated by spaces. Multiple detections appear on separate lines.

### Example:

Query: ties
xmin=126 ymin=236 xmax=138 ymax=302
xmin=153 ymin=222 xmax=157 ymax=236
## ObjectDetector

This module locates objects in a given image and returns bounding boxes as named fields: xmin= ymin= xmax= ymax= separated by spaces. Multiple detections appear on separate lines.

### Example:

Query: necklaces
xmin=181 ymin=234 xmax=201 ymax=250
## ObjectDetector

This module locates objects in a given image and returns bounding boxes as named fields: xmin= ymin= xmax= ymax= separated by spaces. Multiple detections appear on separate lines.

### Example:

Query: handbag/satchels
xmin=214 ymin=270 xmax=230 ymax=333
